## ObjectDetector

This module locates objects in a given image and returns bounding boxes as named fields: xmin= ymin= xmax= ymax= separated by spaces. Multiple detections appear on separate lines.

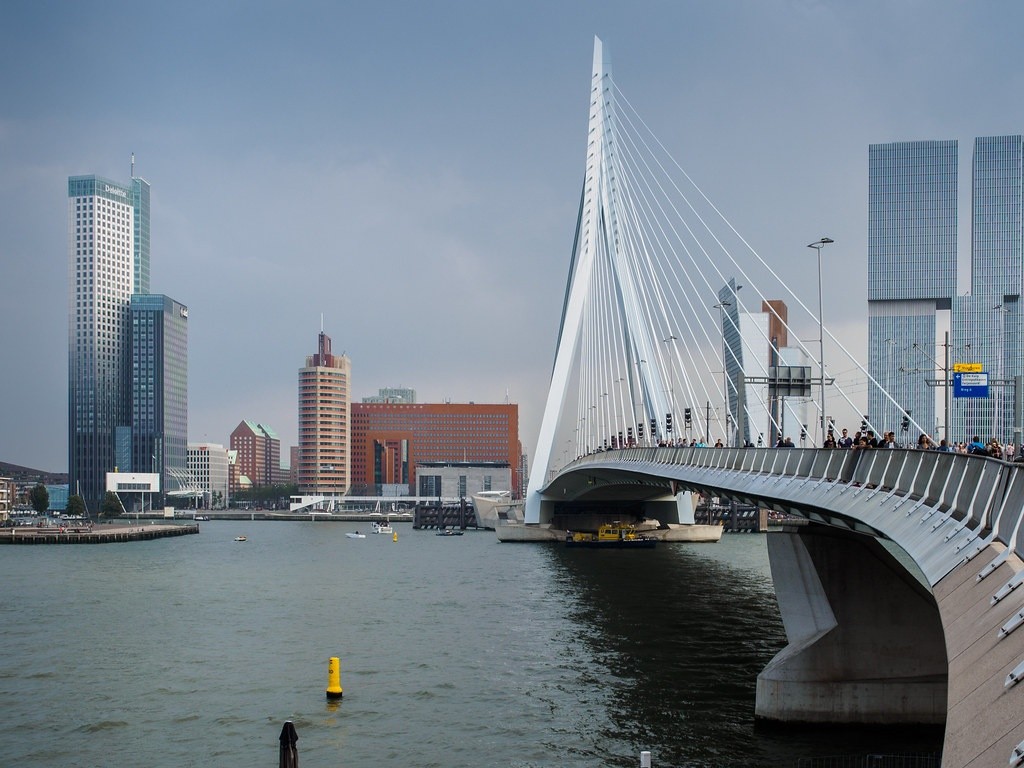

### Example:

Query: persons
xmin=852 ymin=431 xmax=877 ymax=449
xmin=625 ymin=442 xmax=636 ymax=449
xmin=744 ymin=441 xmax=754 ymax=447
xmin=606 ymin=445 xmax=613 ymax=451
xmin=824 ymin=433 xmax=836 ymax=448
xmin=655 ymin=438 xmax=688 ymax=447
xmin=916 ymin=434 xmax=934 ymax=450
xmin=877 ymin=431 xmax=900 ymax=448
xmin=838 ymin=429 xmax=852 ymax=448
xmin=937 ymin=436 xmax=1024 ymax=461
xmin=689 ymin=438 xmax=706 ymax=447
xmin=715 ymin=439 xmax=723 ymax=447
xmin=777 ymin=437 xmax=795 ymax=447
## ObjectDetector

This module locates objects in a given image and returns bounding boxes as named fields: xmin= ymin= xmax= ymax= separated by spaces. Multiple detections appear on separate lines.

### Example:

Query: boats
xmin=565 ymin=519 xmax=663 ymax=547
xmin=194 ymin=515 xmax=209 ymax=521
xmin=436 ymin=528 xmax=465 ymax=536
xmin=370 ymin=519 xmax=393 ymax=534
xmin=344 ymin=530 xmax=367 ymax=539
xmin=235 ymin=536 xmax=246 ymax=541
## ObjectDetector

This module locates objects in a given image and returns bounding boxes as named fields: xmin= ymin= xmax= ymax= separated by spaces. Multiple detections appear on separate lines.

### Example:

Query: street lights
xmin=599 ymin=392 xmax=609 ymax=451
xmin=394 ymin=485 xmax=399 ymax=512
xmin=634 ymin=359 xmax=647 ymax=447
xmin=993 ymin=303 xmax=1012 ymax=446
xmin=614 ymin=377 xmax=626 ymax=448
xmin=805 ymin=237 xmax=835 ymax=449
xmin=712 ymin=300 xmax=732 ymax=446
xmin=662 ymin=336 xmax=678 ymax=447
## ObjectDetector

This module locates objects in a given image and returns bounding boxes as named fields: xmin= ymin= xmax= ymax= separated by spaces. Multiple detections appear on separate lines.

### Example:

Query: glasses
xmin=828 ymin=436 xmax=831 ymax=437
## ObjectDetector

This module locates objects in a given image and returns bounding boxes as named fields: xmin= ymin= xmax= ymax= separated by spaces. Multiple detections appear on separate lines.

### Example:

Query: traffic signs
xmin=952 ymin=371 xmax=990 ymax=399
